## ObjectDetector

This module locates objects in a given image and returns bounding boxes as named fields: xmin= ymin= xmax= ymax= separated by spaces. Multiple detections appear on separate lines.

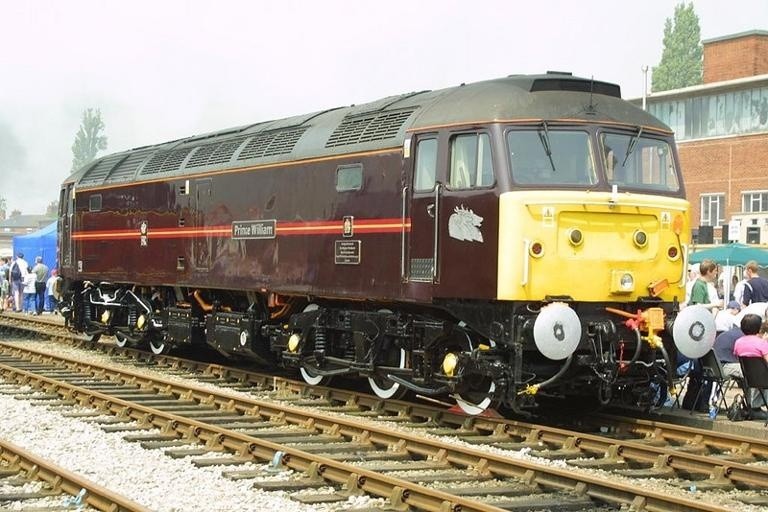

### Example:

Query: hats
xmin=727 ymin=301 xmax=742 ymax=308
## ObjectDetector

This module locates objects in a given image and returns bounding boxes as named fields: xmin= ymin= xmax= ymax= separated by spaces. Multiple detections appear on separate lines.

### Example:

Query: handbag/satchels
xmin=728 ymin=393 xmax=749 ymax=421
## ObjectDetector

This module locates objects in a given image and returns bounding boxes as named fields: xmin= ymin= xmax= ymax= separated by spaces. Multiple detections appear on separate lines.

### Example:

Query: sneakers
xmin=0 ymin=305 xmax=58 ymax=317
xmin=682 ymin=404 xmax=711 ymax=413
xmin=751 ymin=409 xmax=768 ymax=420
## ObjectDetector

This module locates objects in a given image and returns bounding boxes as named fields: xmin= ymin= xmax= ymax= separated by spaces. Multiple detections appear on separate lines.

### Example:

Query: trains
xmin=53 ymin=69 xmax=691 ymax=420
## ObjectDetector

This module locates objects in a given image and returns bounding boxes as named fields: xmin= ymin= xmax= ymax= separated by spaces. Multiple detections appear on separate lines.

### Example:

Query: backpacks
xmin=11 ymin=263 xmax=22 ymax=281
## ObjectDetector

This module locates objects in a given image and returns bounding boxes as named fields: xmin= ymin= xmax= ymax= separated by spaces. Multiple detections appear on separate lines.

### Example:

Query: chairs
xmin=688 ymin=348 xmax=768 ymax=427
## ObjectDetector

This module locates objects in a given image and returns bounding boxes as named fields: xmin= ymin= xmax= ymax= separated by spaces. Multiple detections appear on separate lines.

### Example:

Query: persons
xmin=0 ymin=251 xmax=61 ymax=316
xmin=650 ymin=257 xmax=767 ymax=418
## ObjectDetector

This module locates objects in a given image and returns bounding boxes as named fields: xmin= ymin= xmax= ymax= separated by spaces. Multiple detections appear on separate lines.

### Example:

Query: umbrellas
xmin=688 ymin=239 xmax=768 ymax=305
